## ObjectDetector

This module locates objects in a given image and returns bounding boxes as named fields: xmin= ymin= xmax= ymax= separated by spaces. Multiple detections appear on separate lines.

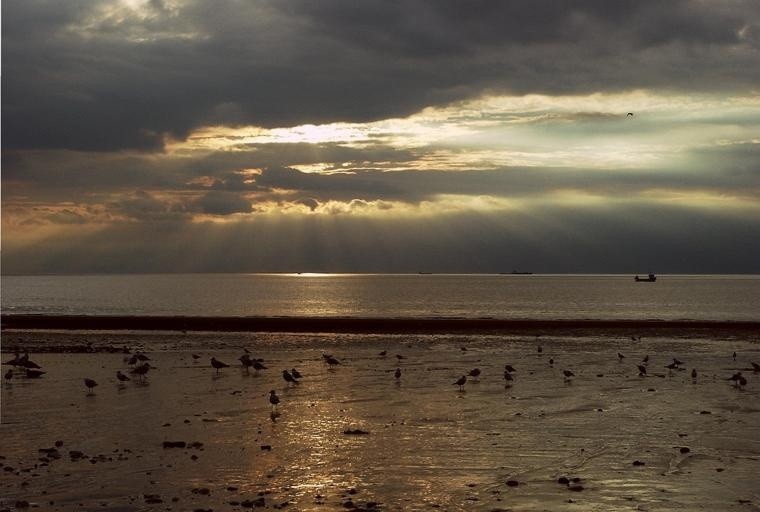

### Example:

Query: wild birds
xmin=116 ymin=345 xmax=158 ymax=387
xmin=395 ymin=368 xmax=401 ymax=379
xmin=269 ymin=390 xmax=280 ymax=408
xmin=190 ymin=348 xmax=305 ymax=385
xmin=378 ymin=350 xmax=387 ymax=356
xmin=323 ymin=354 xmax=341 ymax=368
xmin=83 ymin=377 xmax=100 ymax=393
xmin=1 ymin=352 xmax=48 ymax=385
xmin=451 ymin=336 xmax=760 ymax=390
xmin=396 ymin=354 xmax=408 ymax=362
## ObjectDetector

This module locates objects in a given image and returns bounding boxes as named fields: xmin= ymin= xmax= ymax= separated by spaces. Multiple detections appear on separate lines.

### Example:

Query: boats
xmin=634 ymin=273 xmax=656 ymax=282
xmin=498 ymin=270 xmax=533 ymax=275
